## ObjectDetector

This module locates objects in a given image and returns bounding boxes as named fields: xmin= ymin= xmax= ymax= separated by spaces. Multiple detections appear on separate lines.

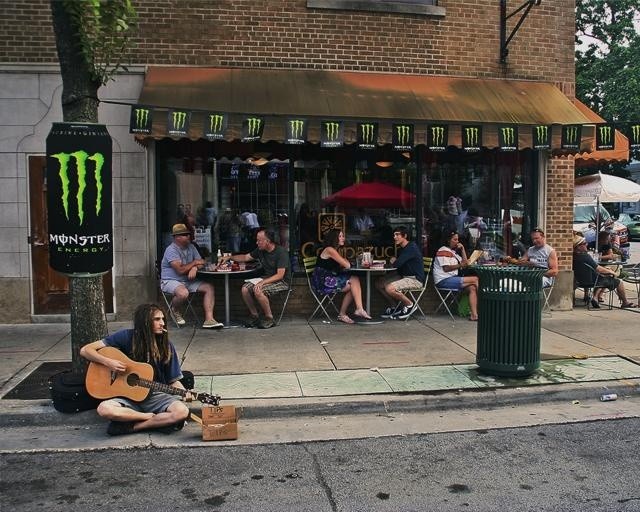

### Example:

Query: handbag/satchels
xmin=458 ymin=294 xmax=471 ymax=316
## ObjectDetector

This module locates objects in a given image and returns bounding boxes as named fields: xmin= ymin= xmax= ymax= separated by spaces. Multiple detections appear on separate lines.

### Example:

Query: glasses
xmin=581 ymin=242 xmax=587 ymax=246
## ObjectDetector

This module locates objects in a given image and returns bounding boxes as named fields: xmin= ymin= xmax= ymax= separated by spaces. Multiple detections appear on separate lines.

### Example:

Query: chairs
xmin=389 ymin=256 xmax=433 ymax=322
xmin=621 ymin=262 xmax=640 ymax=308
xmin=302 ymin=256 xmax=340 ymax=327
xmin=275 ymin=270 xmax=295 ymax=324
xmin=434 ymin=287 xmax=466 ymax=322
xmin=540 ymin=286 xmax=553 ymax=318
xmin=155 ymin=259 xmax=201 ymax=329
xmin=574 ymin=262 xmax=615 ymax=314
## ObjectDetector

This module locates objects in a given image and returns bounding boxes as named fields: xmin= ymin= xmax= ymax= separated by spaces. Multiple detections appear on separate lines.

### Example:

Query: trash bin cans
xmin=469 ymin=264 xmax=549 ymax=378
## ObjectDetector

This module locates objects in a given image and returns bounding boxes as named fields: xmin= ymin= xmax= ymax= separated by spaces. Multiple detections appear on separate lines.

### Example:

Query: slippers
xmin=621 ymin=302 xmax=637 ymax=308
xmin=591 ymin=295 xmax=604 ymax=308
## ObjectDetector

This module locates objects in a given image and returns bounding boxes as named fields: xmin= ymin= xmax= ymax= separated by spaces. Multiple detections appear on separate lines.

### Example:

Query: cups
xmin=204 ymin=257 xmax=212 ymax=271
xmin=239 ymin=265 xmax=245 ymax=271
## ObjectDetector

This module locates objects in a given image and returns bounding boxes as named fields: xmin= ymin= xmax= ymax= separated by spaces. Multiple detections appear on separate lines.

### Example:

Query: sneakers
xmin=381 ymin=306 xmax=402 ymax=317
xmin=108 ymin=422 xmax=134 ymax=436
xmin=171 ymin=309 xmax=186 ymax=325
xmin=337 ymin=314 xmax=354 ymax=324
xmin=258 ymin=316 xmax=276 ymax=329
xmin=354 ymin=310 xmax=372 ymax=320
xmin=202 ymin=319 xmax=224 ymax=329
xmin=399 ymin=302 xmax=418 ymax=319
xmin=245 ymin=315 xmax=261 ymax=326
xmin=165 ymin=419 xmax=185 ymax=433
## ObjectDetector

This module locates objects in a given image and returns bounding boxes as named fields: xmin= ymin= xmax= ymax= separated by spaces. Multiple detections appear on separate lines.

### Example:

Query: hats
xmin=225 ymin=208 xmax=232 ymax=212
xmin=170 ymin=223 xmax=191 ymax=236
xmin=573 ymin=235 xmax=585 ymax=247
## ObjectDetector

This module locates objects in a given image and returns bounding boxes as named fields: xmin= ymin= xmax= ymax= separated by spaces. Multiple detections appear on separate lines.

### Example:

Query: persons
xmin=80 ymin=303 xmax=196 ymax=436
xmin=433 ymin=231 xmax=479 ymax=321
xmin=511 ymin=227 xmax=559 ymax=288
xmin=218 ymin=227 xmax=292 ymax=329
xmin=573 ymin=218 xmax=640 ymax=308
xmin=177 ymin=202 xmax=260 ymax=240
xmin=311 ymin=229 xmax=373 ymax=325
xmin=160 ymin=223 xmax=224 ymax=328
xmin=374 ymin=226 xmax=426 ymax=319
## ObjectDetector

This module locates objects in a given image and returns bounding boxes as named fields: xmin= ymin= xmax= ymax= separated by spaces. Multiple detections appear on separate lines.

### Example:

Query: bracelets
xmin=196 ymin=266 xmax=199 ymax=270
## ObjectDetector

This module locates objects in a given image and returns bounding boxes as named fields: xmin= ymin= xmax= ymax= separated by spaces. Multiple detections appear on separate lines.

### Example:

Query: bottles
xmin=217 ymin=249 xmax=222 ymax=262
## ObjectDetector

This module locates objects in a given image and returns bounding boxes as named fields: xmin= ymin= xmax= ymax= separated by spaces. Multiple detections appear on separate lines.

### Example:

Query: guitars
xmin=86 ymin=347 xmax=221 ymax=405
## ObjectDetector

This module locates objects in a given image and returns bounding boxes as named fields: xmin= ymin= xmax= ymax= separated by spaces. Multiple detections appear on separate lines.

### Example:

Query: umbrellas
xmin=574 ymin=169 xmax=640 ymax=255
xmin=323 ymin=181 xmax=416 ymax=208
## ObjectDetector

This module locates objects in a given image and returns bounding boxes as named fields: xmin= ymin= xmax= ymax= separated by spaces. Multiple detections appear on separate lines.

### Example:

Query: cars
xmin=572 ymin=202 xmax=629 ymax=247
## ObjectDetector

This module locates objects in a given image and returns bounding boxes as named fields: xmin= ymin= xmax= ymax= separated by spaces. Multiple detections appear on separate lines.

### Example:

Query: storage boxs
xmin=190 ymin=403 xmax=244 ymax=441
